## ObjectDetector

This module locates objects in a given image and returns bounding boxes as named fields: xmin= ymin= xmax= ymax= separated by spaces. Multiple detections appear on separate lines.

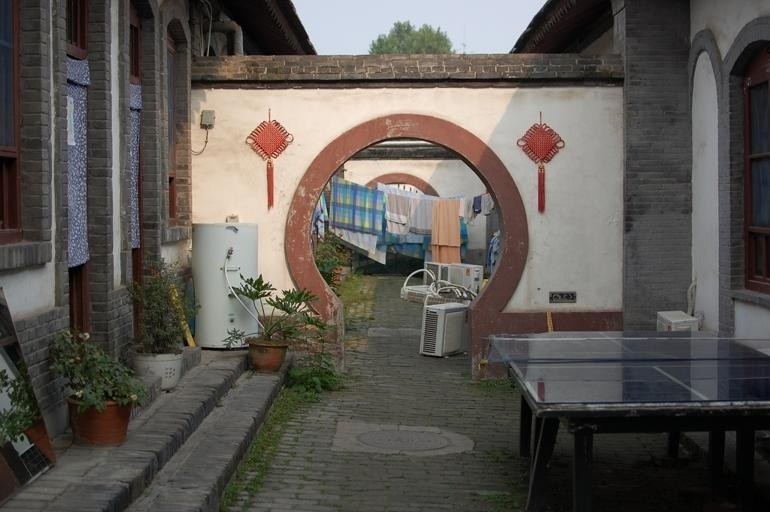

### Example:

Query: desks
xmin=489 ymin=331 xmax=770 ymax=512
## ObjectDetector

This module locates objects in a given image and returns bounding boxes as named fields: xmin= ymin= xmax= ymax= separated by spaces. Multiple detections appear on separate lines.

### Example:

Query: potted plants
xmin=229 ymin=273 xmax=337 ymax=372
xmin=0 ymin=358 xmax=54 ymax=463
xmin=48 ymin=257 xmax=202 ymax=445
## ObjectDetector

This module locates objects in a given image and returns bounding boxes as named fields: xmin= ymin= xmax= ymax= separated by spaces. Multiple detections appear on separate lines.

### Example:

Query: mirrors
xmin=0 ymin=286 xmax=56 ymax=508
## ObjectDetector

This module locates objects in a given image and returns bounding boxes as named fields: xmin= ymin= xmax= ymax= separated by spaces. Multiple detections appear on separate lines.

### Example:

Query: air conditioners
xmin=657 ymin=310 xmax=699 ymax=332
xmin=400 ymin=262 xmax=483 ymax=359
